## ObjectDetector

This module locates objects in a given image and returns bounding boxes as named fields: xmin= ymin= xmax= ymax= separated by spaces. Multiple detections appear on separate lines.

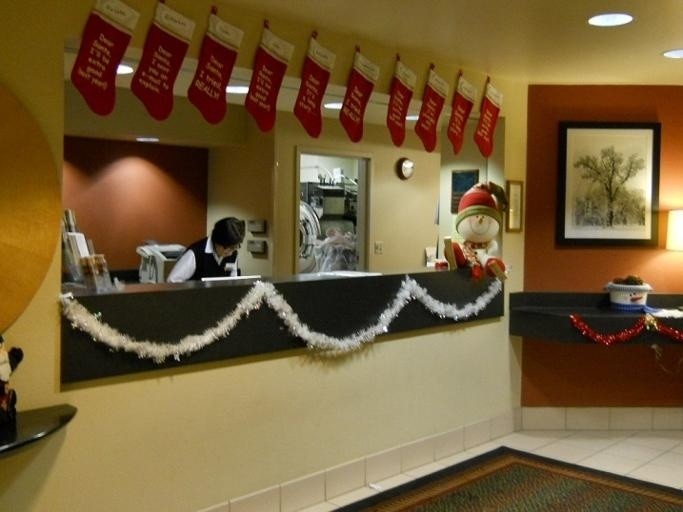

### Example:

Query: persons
xmin=166 ymin=216 xmax=246 ymax=283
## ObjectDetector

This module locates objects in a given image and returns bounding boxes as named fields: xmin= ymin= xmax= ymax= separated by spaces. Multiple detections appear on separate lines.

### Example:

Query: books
xmin=59 ymin=208 xmax=112 ymax=294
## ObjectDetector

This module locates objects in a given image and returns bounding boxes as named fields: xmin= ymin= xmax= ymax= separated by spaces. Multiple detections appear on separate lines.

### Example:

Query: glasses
xmin=223 ymin=244 xmax=241 ymax=251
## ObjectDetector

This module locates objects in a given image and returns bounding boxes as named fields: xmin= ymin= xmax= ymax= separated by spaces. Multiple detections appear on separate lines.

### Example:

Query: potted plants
xmin=605 ymin=275 xmax=653 ymax=312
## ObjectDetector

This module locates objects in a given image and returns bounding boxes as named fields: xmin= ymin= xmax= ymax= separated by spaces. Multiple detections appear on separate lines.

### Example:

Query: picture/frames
xmin=505 ymin=179 xmax=523 ymax=234
xmin=560 ymin=119 xmax=662 ymax=249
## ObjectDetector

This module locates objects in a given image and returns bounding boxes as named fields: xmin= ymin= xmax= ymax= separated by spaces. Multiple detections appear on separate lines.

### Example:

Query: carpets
xmin=324 ymin=443 xmax=682 ymax=512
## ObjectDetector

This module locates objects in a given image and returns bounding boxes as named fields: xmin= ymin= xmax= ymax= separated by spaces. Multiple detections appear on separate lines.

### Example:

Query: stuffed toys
xmin=443 ymin=181 xmax=510 ymax=280
